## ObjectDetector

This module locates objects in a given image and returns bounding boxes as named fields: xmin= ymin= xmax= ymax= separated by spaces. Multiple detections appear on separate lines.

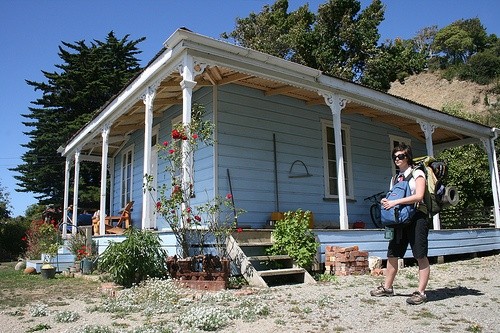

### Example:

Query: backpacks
xmin=381 ymin=172 xmax=417 ymax=227
xmin=411 ymin=155 xmax=458 ymax=217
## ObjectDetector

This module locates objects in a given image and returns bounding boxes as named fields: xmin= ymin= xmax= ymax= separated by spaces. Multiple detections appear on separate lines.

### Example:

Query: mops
xmin=227 ymin=169 xmax=252 ymax=229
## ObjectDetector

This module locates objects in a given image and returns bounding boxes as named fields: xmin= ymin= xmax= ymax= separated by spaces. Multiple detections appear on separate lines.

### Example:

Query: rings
xmin=382 ymin=199 xmax=383 ymax=200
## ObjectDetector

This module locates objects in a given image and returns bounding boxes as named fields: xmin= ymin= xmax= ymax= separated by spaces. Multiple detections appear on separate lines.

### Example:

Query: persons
xmin=370 ymin=144 xmax=430 ymax=305
xmin=81 ymin=207 xmax=88 ymax=214
xmin=68 ymin=204 xmax=73 ymax=211
xmin=92 ymin=210 xmax=107 ymax=223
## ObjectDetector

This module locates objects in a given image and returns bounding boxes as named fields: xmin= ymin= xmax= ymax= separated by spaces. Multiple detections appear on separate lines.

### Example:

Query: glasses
xmin=392 ymin=154 xmax=407 ymax=160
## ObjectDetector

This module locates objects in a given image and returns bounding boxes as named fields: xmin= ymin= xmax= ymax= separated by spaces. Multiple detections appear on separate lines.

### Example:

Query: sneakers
xmin=406 ymin=291 xmax=428 ymax=304
xmin=370 ymin=284 xmax=394 ymax=296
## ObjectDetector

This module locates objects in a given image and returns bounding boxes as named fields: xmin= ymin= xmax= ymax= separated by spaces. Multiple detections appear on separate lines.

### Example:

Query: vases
xmin=75 ymin=261 xmax=84 ymax=272
xmin=41 ymin=265 xmax=57 ymax=278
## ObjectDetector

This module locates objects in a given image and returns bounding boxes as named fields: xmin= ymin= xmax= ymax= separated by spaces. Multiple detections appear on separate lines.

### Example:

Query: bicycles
xmin=364 ymin=191 xmax=386 ymax=228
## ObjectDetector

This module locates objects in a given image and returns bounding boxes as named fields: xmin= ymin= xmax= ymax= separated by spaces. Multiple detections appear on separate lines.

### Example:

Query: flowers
xmin=68 ymin=233 xmax=98 ymax=260
xmin=20 ymin=218 xmax=64 ymax=264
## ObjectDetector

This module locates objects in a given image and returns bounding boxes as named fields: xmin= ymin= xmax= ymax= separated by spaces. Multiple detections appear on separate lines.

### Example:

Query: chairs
xmin=92 ymin=200 xmax=134 ymax=236
